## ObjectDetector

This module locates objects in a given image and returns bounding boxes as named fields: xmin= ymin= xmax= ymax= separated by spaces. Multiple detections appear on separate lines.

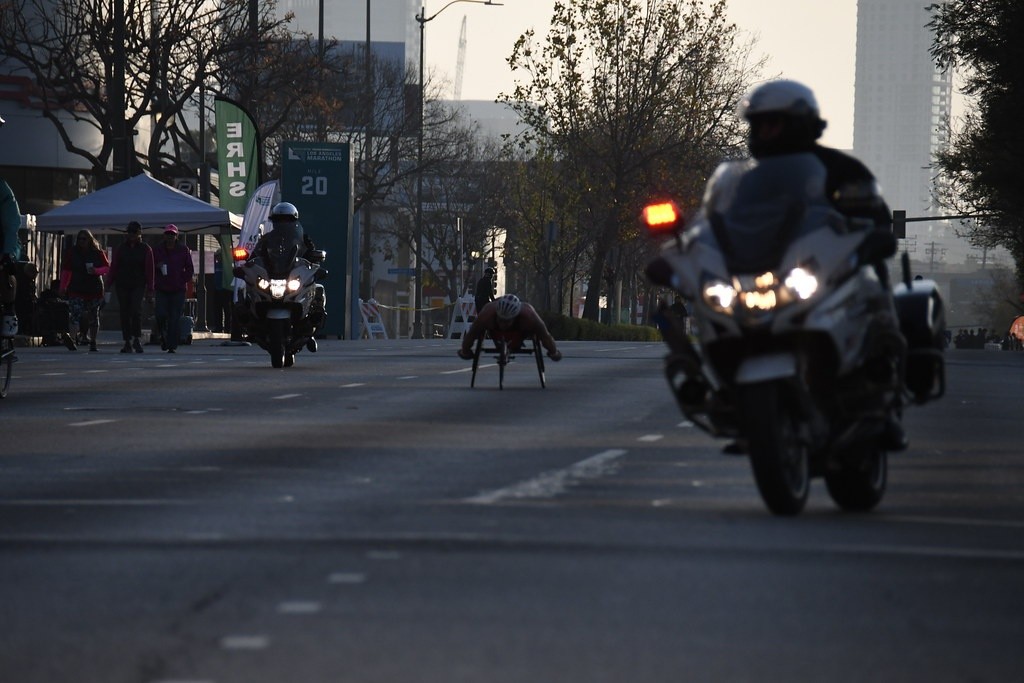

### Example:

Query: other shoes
xmin=132 ymin=338 xmax=143 ymax=353
xmin=159 ymin=337 xmax=167 ymax=351
xmin=307 ymin=336 xmax=318 ymax=352
xmin=120 ymin=344 xmax=133 ymax=353
xmin=882 ymin=410 xmax=911 ymax=453
xmin=167 ymin=349 xmax=175 ymax=353
xmin=62 ymin=332 xmax=78 ymax=351
xmin=90 ymin=340 xmax=97 ymax=351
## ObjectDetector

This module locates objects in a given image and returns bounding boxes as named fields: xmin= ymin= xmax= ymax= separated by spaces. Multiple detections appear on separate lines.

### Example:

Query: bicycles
xmin=0 ymin=252 xmax=19 ymax=398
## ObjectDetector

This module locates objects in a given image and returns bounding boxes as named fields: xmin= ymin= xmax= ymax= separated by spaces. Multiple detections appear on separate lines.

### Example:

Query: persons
xmin=954 ymin=328 xmax=1022 ymax=351
xmin=39 ymin=280 xmax=61 ymax=347
xmin=151 ymin=224 xmax=194 ymax=353
xmin=645 ymin=81 xmax=910 ymax=455
xmin=234 ymin=202 xmax=325 ymax=351
xmin=0 ymin=178 xmax=21 ymax=337
xmin=457 ymin=294 xmax=562 ymax=363
xmin=105 ymin=221 xmax=154 ymax=353
xmin=671 ymin=296 xmax=687 ymax=332
xmin=475 ymin=267 xmax=495 ymax=315
xmin=58 ymin=229 xmax=110 ymax=352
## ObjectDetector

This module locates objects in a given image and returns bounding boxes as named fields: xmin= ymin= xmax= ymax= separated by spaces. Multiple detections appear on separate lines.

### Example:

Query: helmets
xmin=483 ymin=267 xmax=496 ymax=274
xmin=268 ymin=202 xmax=299 ymax=222
xmin=742 ymin=77 xmax=821 ymax=120
xmin=495 ymin=293 xmax=522 ymax=320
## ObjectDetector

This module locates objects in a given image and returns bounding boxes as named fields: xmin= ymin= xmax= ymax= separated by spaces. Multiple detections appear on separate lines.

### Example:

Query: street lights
xmin=456 ymin=217 xmax=463 ymax=297
xmin=411 ymin=0 xmax=504 ymax=339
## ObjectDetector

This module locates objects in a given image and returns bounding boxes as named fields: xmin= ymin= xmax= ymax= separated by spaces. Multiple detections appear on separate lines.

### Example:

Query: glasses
xmin=78 ymin=236 xmax=87 ymax=240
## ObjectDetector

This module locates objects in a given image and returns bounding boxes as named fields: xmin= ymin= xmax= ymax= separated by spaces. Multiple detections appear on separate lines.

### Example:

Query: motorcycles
xmin=643 ymin=199 xmax=947 ymax=513
xmin=232 ymin=248 xmax=328 ymax=368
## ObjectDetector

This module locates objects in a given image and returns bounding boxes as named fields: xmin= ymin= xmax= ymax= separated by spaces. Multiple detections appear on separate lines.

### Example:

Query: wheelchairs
xmin=458 ymin=327 xmax=551 ymax=390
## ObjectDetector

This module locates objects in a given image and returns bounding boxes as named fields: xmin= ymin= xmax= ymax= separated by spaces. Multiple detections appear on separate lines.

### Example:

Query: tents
xmin=33 ymin=174 xmax=242 ymax=345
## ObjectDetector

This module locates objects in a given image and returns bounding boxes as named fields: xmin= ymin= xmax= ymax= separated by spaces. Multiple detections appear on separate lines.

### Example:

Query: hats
xmin=126 ymin=221 xmax=142 ymax=233
xmin=163 ymin=224 xmax=179 ymax=234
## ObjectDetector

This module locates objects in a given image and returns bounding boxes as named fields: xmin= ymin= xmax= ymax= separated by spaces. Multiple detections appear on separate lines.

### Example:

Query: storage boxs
xmin=893 ymin=278 xmax=950 ymax=403
xmin=312 ymin=284 xmax=327 ymax=325
